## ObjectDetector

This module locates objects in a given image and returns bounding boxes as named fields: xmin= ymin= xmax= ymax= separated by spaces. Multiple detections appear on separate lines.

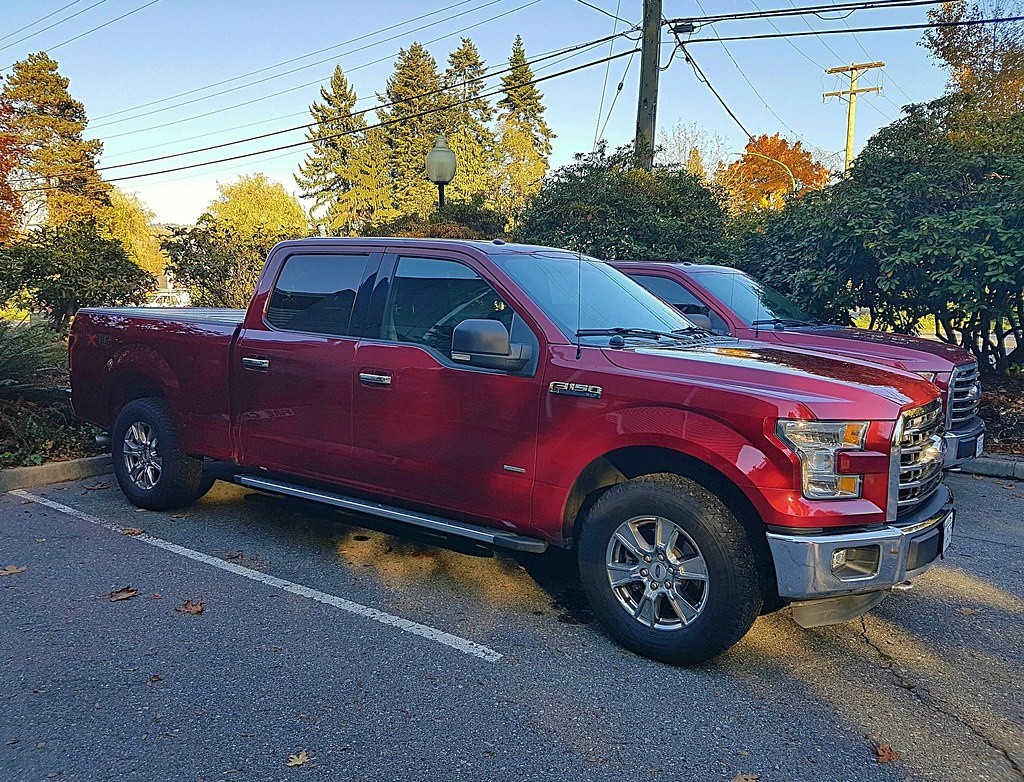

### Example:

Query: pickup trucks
xmin=64 ymin=235 xmax=959 ymax=666
xmin=600 ymin=259 xmax=991 ymax=463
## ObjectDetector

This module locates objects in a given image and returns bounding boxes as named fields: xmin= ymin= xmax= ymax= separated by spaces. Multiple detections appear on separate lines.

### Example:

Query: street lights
xmin=421 ymin=136 xmax=459 ymax=216
